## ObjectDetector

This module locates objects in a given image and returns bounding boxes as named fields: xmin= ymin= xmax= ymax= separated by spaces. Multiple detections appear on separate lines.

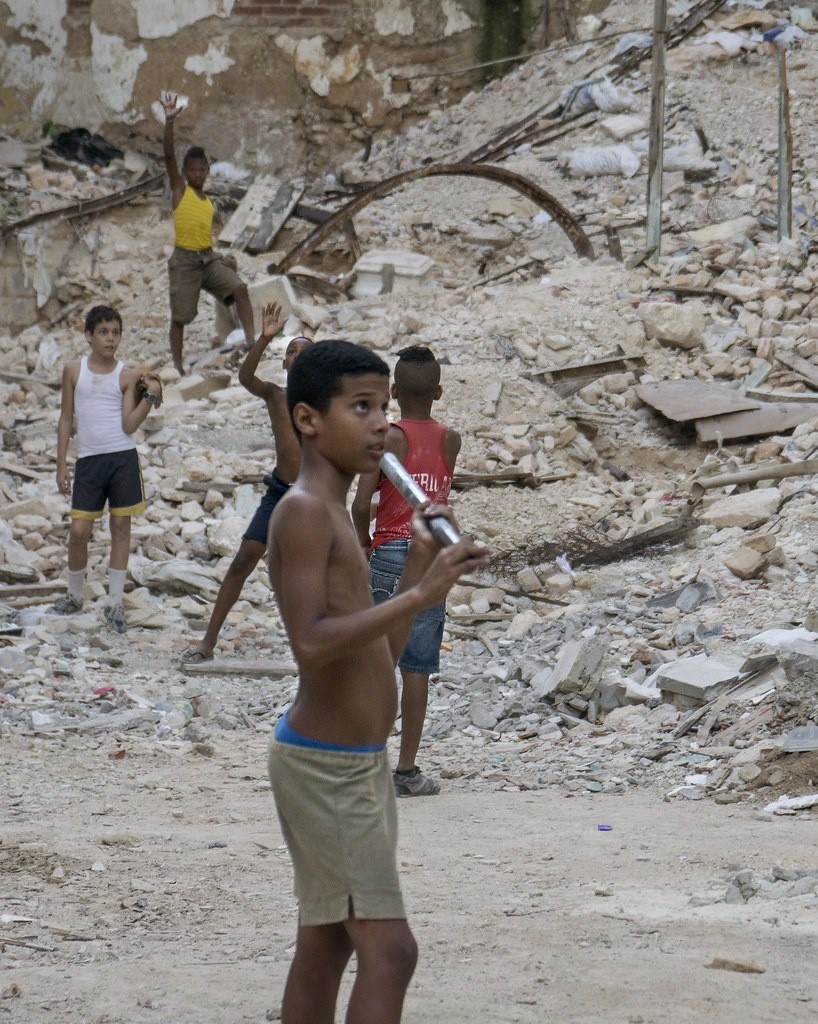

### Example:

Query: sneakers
xmin=44 ymin=593 xmax=84 ymax=615
xmin=392 ymin=766 xmax=440 ymax=798
xmin=101 ymin=604 xmax=127 ymax=635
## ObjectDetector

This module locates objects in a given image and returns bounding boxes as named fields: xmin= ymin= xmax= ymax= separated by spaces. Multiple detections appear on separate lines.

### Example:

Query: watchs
xmin=144 ymin=392 xmax=157 ymax=403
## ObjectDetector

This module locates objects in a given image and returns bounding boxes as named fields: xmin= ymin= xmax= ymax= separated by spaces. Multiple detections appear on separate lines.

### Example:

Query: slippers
xmin=180 ymin=651 xmax=214 ymax=664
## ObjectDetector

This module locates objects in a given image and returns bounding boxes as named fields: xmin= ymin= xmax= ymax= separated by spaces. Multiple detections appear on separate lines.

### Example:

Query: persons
xmin=44 ymin=305 xmax=160 ymax=632
xmin=353 ymin=344 xmax=462 ymax=797
xmin=157 ymin=93 xmax=256 ymax=373
xmin=270 ymin=340 xmax=492 ymax=1024
xmin=184 ymin=301 xmax=312 ymax=666
